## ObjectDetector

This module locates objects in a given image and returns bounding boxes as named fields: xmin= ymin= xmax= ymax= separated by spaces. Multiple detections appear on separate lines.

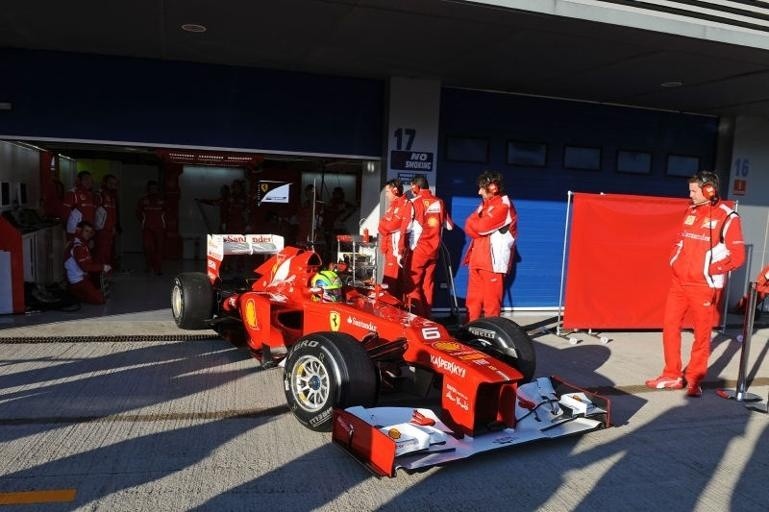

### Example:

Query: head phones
xmin=699 ymin=175 xmax=719 ymax=206
xmin=392 ymin=182 xmax=399 ymax=196
xmin=487 ymin=177 xmax=499 ymax=195
xmin=101 ymin=175 xmax=106 ymax=189
xmin=75 ymin=171 xmax=96 ymax=185
xmin=412 ymin=180 xmax=420 ymax=194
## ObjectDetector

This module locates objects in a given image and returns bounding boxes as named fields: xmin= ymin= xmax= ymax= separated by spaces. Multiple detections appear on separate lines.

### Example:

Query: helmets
xmin=311 ymin=271 xmax=344 ymax=302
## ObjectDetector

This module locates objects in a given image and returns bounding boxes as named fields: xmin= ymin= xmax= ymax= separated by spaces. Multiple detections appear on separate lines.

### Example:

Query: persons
xmin=730 ymin=263 xmax=769 ymax=312
xmin=462 ymin=167 xmax=518 ymax=321
xmin=401 ymin=175 xmax=454 ymax=320
xmin=55 ymin=170 xmax=345 ymax=305
xmin=645 ymin=170 xmax=746 ymax=397
xmin=378 ymin=178 xmax=415 ymax=297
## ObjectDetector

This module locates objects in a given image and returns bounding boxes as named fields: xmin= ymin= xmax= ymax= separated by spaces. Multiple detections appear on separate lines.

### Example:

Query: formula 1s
xmin=169 ymin=229 xmax=616 ymax=481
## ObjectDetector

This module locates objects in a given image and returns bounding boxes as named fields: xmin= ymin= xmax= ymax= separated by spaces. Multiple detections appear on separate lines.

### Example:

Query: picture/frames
xmin=444 ymin=133 xmax=701 ymax=179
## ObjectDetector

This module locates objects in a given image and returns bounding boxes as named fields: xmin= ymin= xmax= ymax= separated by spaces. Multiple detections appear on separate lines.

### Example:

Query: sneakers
xmin=687 ymin=382 xmax=703 ymax=397
xmin=646 ymin=376 xmax=683 ymax=389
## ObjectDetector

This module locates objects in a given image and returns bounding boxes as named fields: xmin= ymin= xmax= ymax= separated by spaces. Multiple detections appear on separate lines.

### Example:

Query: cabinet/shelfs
xmin=336 ymin=235 xmax=378 ymax=290
